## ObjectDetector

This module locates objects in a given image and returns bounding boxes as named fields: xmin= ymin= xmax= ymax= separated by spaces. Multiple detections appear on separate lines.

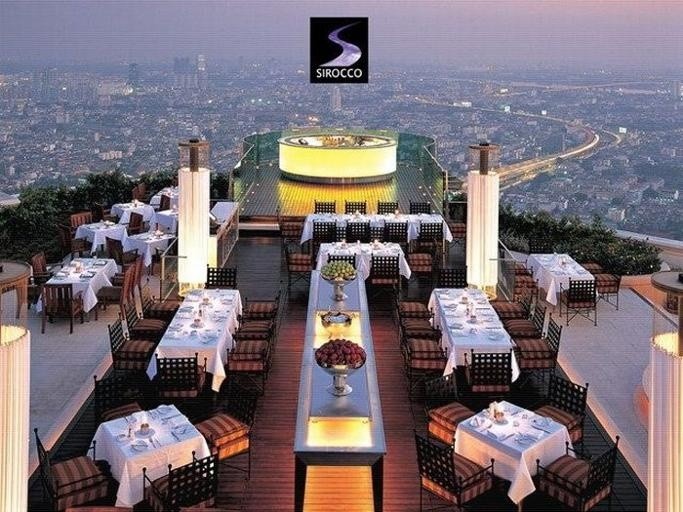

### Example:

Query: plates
xmin=116 ymin=403 xmax=192 ymax=453
xmin=467 ymin=400 xmax=552 ymax=448
xmin=166 ymin=287 xmax=236 ymax=341
xmin=437 ymin=287 xmax=505 ymax=342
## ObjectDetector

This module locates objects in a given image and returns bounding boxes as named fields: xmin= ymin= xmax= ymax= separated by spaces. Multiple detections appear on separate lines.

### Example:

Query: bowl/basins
xmin=320 ymin=267 xmax=357 ymax=285
xmin=321 ymin=312 xmax=352 ymax=330
xmin=314 ymin=346 xmax=366 ymax=378
